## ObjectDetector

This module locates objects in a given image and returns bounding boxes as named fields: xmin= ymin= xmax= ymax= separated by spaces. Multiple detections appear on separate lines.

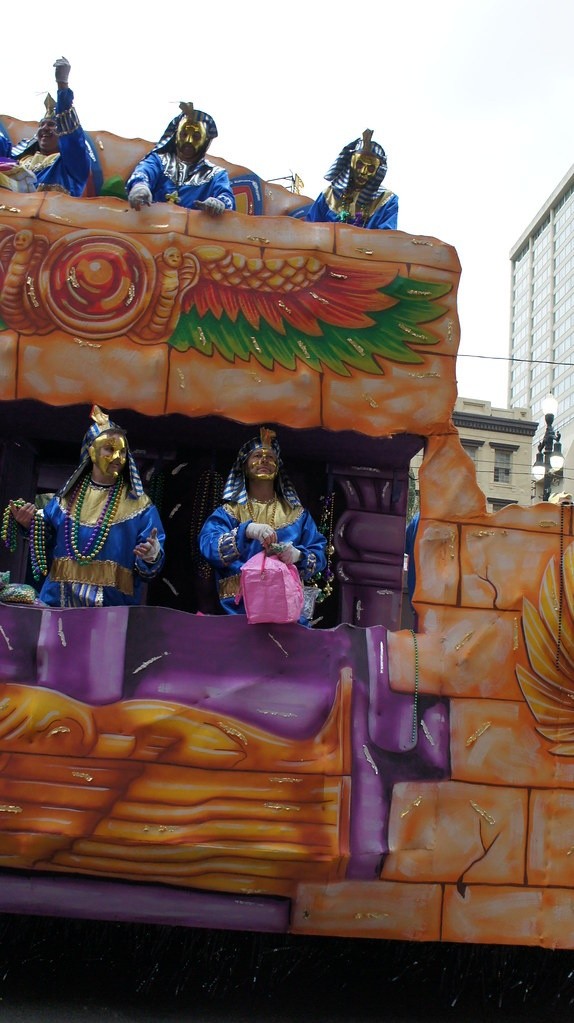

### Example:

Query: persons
xmin=8 ymin=420 xmax=168 ymax=612
xmin=0 ymin=55 xmax=92 ymax=196
xmin=138 ymin=417 xmax=227 ymax=627
xmin=199 ymin=435 xmax=328 ymax=621
xmin=307 ymin=140 xmax=397 ymax=231
xmin=124 ymin=110 xmax=233 ymax=217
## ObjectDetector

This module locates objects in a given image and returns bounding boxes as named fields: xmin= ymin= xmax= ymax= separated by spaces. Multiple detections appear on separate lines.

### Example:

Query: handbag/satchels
xmin=240 ymin=547 xmax=304 ymax=624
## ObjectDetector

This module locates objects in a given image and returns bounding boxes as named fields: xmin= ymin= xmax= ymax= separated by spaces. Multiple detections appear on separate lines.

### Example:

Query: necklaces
xmin=144 ymin=464 xmax=167 ymax=513
xmin=189 ymin=468 xmax=227 ymax=579
xmin=165 ymin=157 xmax=194 ymax=204
xmin=340 ymin=193 xmax=370 ymax=226
xmin=62 ymin=459 xmax=126 ymax=566
xmin=2 ymin=493 xmax=50 ymax=585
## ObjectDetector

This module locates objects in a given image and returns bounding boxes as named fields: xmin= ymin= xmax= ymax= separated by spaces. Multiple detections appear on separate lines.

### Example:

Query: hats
xmin=222 ymin=437 xmax=302 ymax=509
xmin=138 ymin=101 xmax=218 ymax=176
xmin=11 ymin=93 xmax=57 ymax=157
xmin=324 ymin=128 xmax=387 ymax=207
xmin=57 ymin=404 xmax=144 ymax=497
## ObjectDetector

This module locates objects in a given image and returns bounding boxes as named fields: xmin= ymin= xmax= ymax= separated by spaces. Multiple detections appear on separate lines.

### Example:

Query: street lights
xmin=528 ymin=393 xmax=565 ymax=505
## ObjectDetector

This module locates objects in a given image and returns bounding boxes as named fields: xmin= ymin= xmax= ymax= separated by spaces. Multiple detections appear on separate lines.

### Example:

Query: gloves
xmin=128 ymin=182 xmax=152 ymax=212
xmin=53 ymin=56 xmax=71 ymax=84
xmin=194 ymin=196 xmax=226 ymax=216
